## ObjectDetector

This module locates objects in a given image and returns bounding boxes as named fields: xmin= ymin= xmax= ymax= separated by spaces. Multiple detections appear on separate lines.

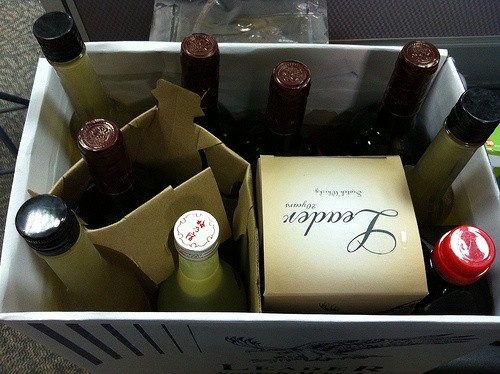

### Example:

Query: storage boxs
xmin=0 ymin=41 xmax=500 ymax=374
xmin=257 ymin=152 xmax=428 ymax=314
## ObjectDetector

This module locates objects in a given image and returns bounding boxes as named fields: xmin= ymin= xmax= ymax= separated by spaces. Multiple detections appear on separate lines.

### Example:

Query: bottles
xmin=403 ymin=87 xmax=500 ymax=230
xmin=179 ymin=32 xmax=240 ymax=153
xmin=32 ymin=11 xmax=133 ymax=150
xmin=394 ymin=226 xmax=497 ymax=315
xmin=333 ymin=39 xmax=441 ymax=166
xmin=241 ymin=60 xmax=324 ymax=166
xmin=155 ymin=209 xmax=246 ymax=311
xmin=75 ymin=118 xmax=152 ymax=224
xmin=14 ymin=194 xmax=154 ymax=313
